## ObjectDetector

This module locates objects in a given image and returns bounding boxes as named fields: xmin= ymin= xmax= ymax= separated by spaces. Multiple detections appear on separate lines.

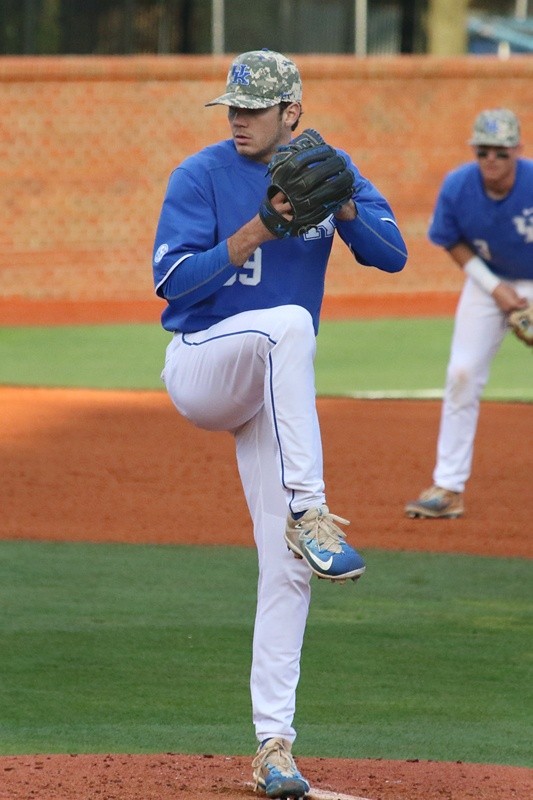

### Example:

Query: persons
xmin=402 ymin=108 xmax=533 ymax=521
xmin=153 ymin=47 xmax=408 ymax=798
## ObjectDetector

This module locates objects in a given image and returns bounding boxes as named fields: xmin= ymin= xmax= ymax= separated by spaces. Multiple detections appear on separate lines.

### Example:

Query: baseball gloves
xmin=259 ymin=128 xmax=356 ymax=238
xmin=508 ymin=304 xmax=533 ymax=349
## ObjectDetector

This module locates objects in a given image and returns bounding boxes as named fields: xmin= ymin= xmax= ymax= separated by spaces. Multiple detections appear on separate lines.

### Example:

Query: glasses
xmin=476 ymin=150 xmax=512 ymax=160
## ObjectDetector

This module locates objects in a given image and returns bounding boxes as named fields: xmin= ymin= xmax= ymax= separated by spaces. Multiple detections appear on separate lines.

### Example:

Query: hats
xmin=470 ymin=109 xmax=519 ymax=147
xmin=204 ymin=48 xmax=303 ymax=109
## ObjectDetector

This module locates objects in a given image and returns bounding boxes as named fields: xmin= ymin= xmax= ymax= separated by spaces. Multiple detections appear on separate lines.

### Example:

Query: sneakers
xmin=284 ymin=505 xmax=366 ymax=584
xmin=252 ymin=736 xmax=310 ymax=797
xmin=405 ymin=486 xmax=464 ymax=519
